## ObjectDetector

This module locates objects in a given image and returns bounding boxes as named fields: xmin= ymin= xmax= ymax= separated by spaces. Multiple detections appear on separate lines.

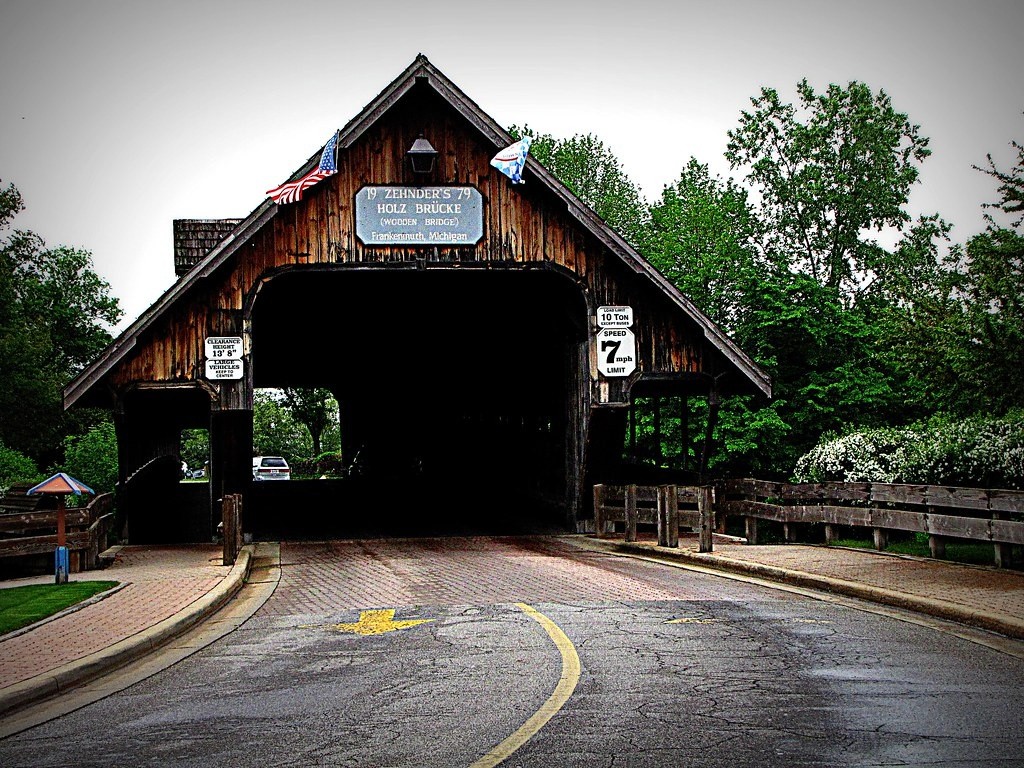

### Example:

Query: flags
xmin=490 ymin=135 xmax=533 ymax=184
xmin=266 ymin=135 xmax=337 ymax=205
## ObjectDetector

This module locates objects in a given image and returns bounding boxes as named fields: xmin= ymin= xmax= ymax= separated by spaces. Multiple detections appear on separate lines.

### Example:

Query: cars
xmin=252 ymin=456 xmax=290 ymax=481
xmin=346 ymin=443 xmax=425 ymax=493
